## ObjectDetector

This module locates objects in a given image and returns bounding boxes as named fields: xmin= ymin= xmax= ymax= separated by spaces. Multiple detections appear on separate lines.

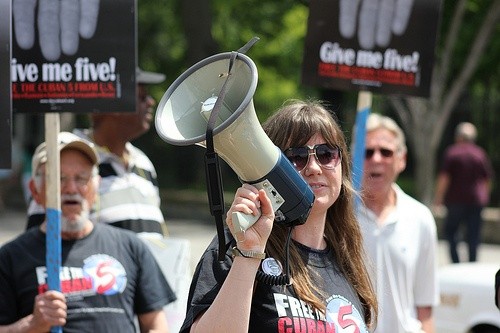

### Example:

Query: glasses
xmin=364 ymin=148 xmax=397 ymax=161
xmin=36 ymin=171 xmax=94 ymax=188
xmin=282 ymin=142 xmax=344 ymax=174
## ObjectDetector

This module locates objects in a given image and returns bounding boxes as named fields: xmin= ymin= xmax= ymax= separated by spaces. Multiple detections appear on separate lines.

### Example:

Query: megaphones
xmin=155 ymin=51 xmax=315 ymax=233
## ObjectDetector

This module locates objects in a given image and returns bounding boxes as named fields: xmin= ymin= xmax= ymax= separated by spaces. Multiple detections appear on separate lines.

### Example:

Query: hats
xmin=31 ymin=131 xmax=101 ymax=177
xmin=135 ymin=63 xmax=168 ymax=85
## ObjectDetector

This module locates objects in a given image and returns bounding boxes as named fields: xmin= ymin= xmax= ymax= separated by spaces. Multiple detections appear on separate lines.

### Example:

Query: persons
xmin=351 ymin=113 xmax=441 ymax=333
xmin=26 ymin=67 xmax=169 ymax=261
xmin=178 ymin=99 xmax=378 ymax=333
xmin=0 ymin=131 xmax=177 ymax=333
xmin=432 ymin=122 xmax=494 ymax=263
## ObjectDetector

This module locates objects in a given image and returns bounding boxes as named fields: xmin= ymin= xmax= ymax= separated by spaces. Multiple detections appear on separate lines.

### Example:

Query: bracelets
xmin=231 ymin=246 xmax=266 ymax=259
